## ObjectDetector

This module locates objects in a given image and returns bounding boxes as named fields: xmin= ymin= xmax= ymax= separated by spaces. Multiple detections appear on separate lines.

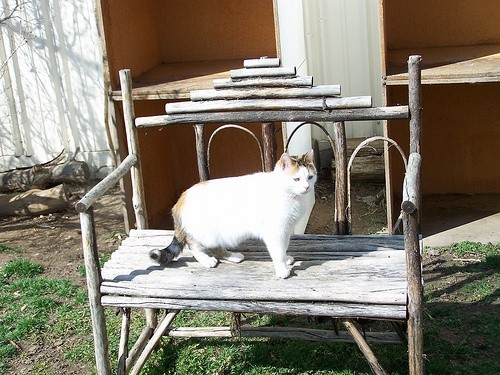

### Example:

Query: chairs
xmin=73 ymin=53 xmax=427 ymax=374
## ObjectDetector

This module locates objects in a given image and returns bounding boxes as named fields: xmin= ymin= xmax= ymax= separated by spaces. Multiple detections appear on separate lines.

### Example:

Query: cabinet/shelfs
xmin=96 ymin=0 xmax=317 ymax=235
xmin=379 ymin=0 xmax=500 ymax=255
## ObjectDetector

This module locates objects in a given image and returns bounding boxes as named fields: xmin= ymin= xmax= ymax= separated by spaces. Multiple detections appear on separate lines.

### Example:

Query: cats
xmin=148 ymin=149 xmax=318 ymax=279
